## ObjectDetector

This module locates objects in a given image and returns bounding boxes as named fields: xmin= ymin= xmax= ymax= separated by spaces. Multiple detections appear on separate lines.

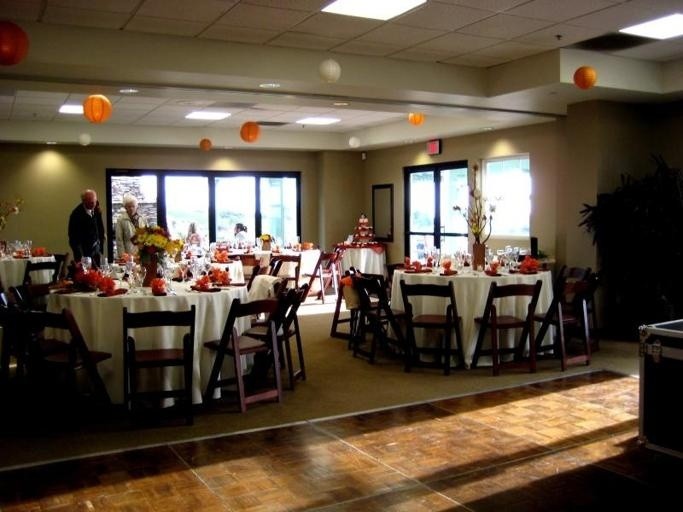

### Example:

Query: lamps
xmin=317 ymin=57 xmax=341 ymax=85
xmin=348 ymin=136 xmax=361 ymax=148
xmin=200 ymin=138 xmax=211 ymax=151
xmin=78 ymin=132 xmax=91 ymax=147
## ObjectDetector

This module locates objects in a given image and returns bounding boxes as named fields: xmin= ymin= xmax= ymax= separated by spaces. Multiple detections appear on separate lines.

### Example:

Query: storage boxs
xmin=637 ymin=320 xmax=683 ymax=459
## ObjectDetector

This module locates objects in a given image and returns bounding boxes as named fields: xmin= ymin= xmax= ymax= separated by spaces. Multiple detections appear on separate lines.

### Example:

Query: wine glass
xmin=0 ymin=239 xmax=32 ymax=260
xmin=416 ymin=239 xmax=527 ymax=276
xmin=81 ymin=252 xmax=210 ymax=293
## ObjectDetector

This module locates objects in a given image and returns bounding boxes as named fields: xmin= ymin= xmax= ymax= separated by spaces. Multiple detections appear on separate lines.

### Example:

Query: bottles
xmin=292 ymin=242 xmax=314 ymax=252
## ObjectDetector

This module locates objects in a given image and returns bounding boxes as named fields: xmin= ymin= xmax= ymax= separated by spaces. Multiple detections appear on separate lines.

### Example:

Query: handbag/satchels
xmin=342 ymin=277 xmax=369 ymax=309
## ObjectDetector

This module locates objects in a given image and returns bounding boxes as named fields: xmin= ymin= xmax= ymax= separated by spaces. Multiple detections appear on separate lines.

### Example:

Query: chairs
xmin=330 ymin=264 xmax=602 ymax=376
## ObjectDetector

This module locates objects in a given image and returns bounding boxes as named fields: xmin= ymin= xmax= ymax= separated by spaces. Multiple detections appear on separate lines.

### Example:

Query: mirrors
xmin=371 ymin=183 xmax=393 ymax=242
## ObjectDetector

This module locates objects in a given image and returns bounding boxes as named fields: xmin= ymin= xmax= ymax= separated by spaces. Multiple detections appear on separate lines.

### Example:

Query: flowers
xmin=128 ymin=225 xmax=186 ymax=268
xmin=466 ymin=160 xmax=490 ymax=245
xmin=0 ymin=195 xmax=26 ymax=234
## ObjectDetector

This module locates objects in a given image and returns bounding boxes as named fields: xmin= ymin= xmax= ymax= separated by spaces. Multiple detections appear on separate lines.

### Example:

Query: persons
xmin=115 ymin=193 xmax=148 ymax=260
xmin=232 ymin=223 xmax=249 ymax=240
xmin=66 ymin=186 xmax=105 ymax=270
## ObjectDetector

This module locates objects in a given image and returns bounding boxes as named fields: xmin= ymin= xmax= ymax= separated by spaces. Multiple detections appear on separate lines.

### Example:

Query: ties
xmin=90 ymin=210 xmax=95 ymax=217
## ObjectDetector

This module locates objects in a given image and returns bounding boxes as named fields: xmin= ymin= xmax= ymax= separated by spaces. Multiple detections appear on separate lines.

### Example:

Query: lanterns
xmin=199 ymin=139 xmax=210 ymax=151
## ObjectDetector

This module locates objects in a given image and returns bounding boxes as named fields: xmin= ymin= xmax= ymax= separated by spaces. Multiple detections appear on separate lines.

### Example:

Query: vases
xmin=472 ymin=244 xmax=485 ymax=271
